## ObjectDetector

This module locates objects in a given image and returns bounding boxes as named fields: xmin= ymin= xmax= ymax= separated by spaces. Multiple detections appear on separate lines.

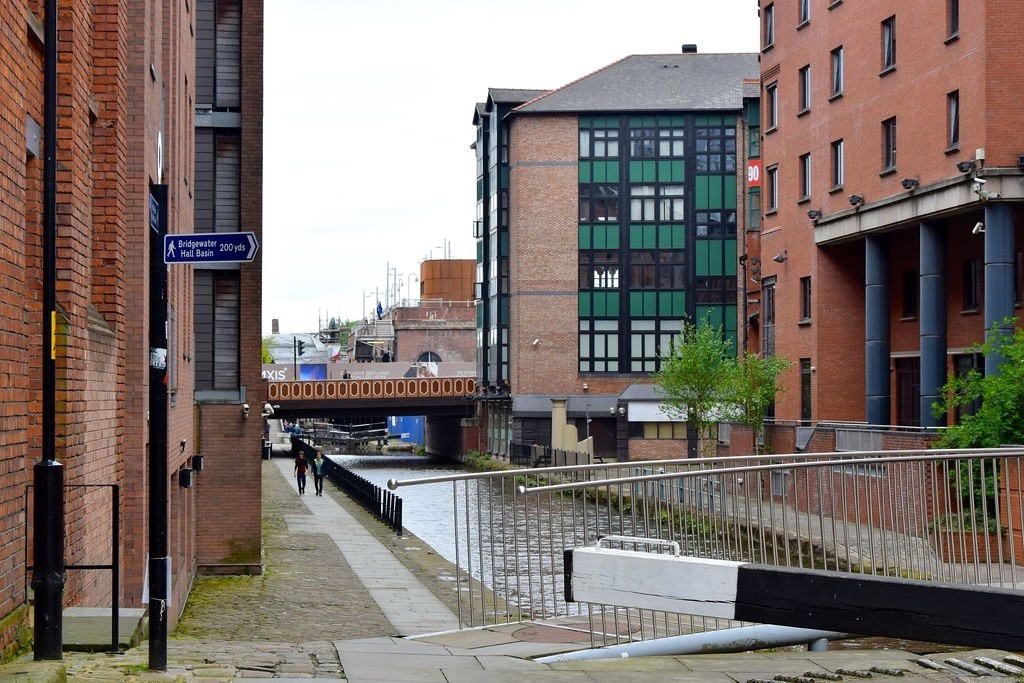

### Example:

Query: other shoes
xmin=316 ymin=491 xmax=318 ymax=495
xmin=301 ymin=487 xmax=305 ymax=494
xmin=319 ymin=493 xmax=322 ymax=496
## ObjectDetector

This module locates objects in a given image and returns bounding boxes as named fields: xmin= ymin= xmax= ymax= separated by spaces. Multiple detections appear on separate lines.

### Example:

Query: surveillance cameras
xmin=973 ymin=178 xmax=987 ymax=185
xmin=243 ymin=404 xmax=250 ymax=412
xmin=973 ymin=222 xmax=983 ymax=234
xmin=532 ymin=338 xmax=539 ymax=346
xmin=265 ymin=404 xmax=274 ymax=415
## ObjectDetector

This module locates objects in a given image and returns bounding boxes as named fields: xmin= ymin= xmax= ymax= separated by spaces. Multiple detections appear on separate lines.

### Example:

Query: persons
xmin=341 ymin=370 xmax=352 ymax=379
xmin=293 ymin=451 xmax=308 ymax=497
xmin=311 ymin=451 xmax=328 ymax=497
xmin=363 ymin=320 xmax=369 ymax=335
xmin=283 ymin=419 xmax=300 ymax=434
xmin=381 ymin=352 xmax=390 ymax=362
xmin=377 ymin=302 xmax=384 ymax=320
